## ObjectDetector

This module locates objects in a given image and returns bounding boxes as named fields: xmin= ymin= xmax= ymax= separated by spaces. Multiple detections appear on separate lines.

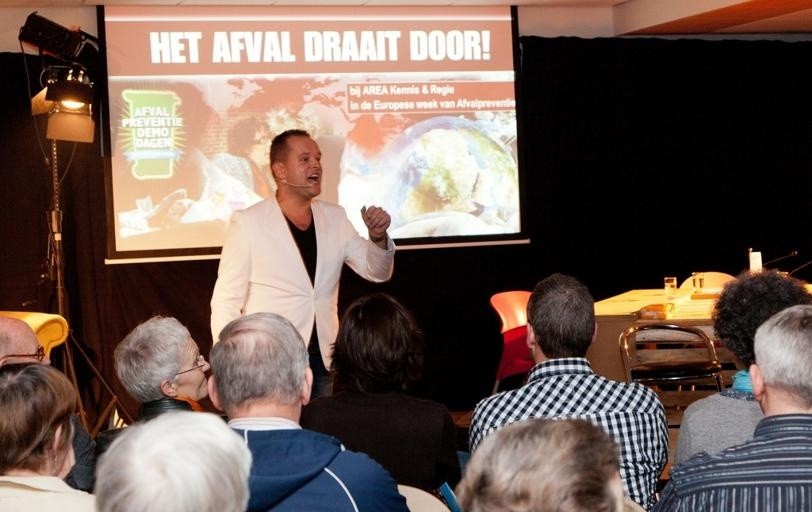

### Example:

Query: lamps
xmin=40 ymin=60 xmax=95 ymax=113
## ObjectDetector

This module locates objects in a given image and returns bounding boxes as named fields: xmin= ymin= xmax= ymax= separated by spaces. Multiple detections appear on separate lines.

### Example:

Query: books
xmin=635 ymin=302 xmax=674 ymax=318
xmin=692 ymin=289 xmax=722 ymax=299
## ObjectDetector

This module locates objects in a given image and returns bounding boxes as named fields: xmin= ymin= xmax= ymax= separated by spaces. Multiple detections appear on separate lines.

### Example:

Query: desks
xmin=587 ymin=282 xmax=812 ymax=427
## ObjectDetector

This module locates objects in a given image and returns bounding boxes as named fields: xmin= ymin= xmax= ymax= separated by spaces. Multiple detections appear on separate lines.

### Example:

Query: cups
xmin=665 ymin=277 xmax=678 ymax=300
xmin=691 ymin=272 xmax=707 ymax=296
xmin=664 ymin=302 xmax=681 ymax=319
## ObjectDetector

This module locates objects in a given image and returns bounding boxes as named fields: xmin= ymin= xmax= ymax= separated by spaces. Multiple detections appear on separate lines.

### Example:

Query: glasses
xmin=1 ymin=347 xmax=45 ymax=361
xmin=174 ymin=354 xmax=206 ymax=377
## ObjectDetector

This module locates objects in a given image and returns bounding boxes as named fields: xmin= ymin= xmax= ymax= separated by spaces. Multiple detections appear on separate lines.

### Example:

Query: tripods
xmin=45 ymin=141 xmax=136 ymax=441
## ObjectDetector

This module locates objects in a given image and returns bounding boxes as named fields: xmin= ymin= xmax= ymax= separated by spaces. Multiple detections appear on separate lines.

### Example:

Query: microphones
xmin=786 ymin=260 xmax=812 ymax=277
xmin=281 ymin=178 xmax=315 ymax=189
xmin=762 ymin=247 xmax=799 ymax=268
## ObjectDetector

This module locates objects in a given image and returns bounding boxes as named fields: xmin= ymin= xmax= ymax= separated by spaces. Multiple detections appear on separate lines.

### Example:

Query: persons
xmin=208 ymin=312 xmax=411 ymax=512
xmin=648 ymin=304 xmax=811 ymax=510
xmin=457 ymin=417 xmax=624 ymax=512
xmin=0 ymin=361 xmax=96 ymax=511
xmin=212 ymin=129 xmax=395 ymax=395
xmin=94 ymin=408 xmax=252 ymax=512
xmin=95 ymin=316 xmax=213 ymax=452
xmin=300 ymin=298 xmax=460 ymax=493
xmin=673 ymin=271 xmax=808 ymax=460
xmin=1 ymin=315 xmax=97 ymax=494
xmin=469 ymin=274 xmax=669 ymax=511
xmin=215 ymin=117 xmax=277 ymax=200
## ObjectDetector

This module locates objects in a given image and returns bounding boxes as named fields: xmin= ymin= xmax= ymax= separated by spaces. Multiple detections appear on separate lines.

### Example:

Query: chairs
xmin=678 ymin=271 xmax=738 ymax=288
xmin=490 ymin=289 xmax=537 ymax=395
xmin=618 ymin=320 xmax=727 ymax=430
